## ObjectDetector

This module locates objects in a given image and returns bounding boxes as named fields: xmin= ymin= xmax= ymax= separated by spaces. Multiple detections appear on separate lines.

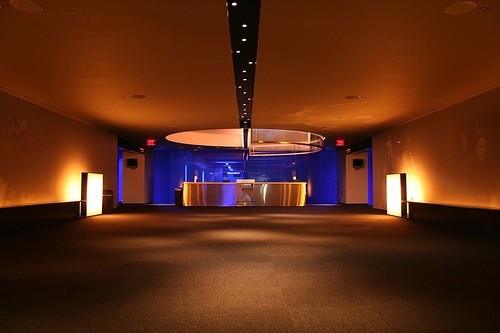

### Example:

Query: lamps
xmin=81 ymin=172 xmax=104 ymax=217
xmin=385 ymin=173 xmax=407 ymax=217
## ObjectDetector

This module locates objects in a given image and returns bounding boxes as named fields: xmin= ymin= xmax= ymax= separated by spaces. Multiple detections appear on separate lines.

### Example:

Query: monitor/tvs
xmin=353 ymin=158 xmax=364 ymax=168
xmin=127 ymin=159 xmax=138 ymax=168
